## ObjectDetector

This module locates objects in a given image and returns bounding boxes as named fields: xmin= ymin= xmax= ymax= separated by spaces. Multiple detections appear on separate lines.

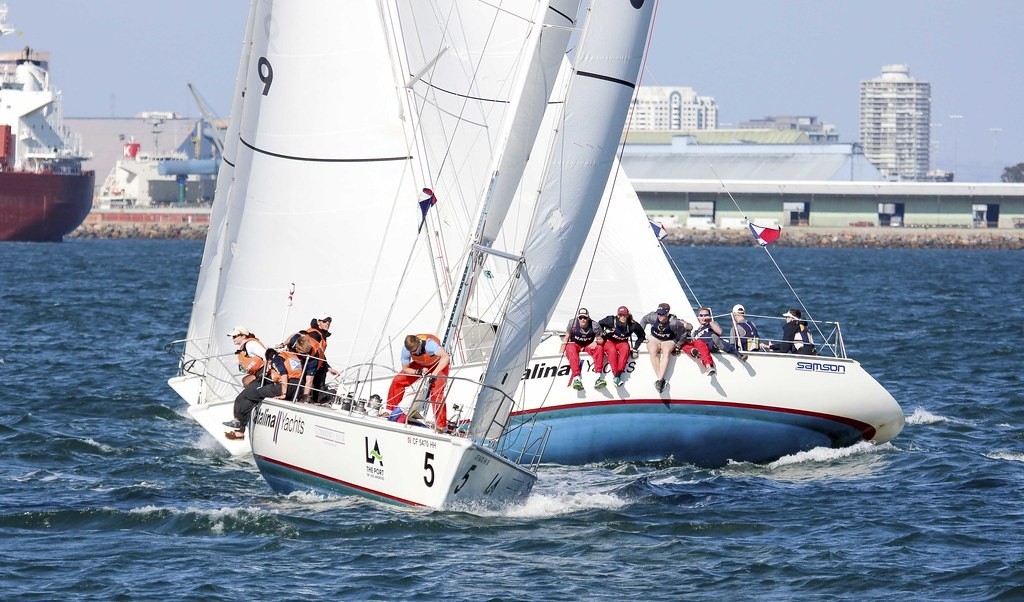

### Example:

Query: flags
xmin=750 ymin=221 xmax=781 ymax=247
xmin=417 ymin=188 xmax=437 ymax=234
xmin=648 ymin=219 xmax=668 ymax=241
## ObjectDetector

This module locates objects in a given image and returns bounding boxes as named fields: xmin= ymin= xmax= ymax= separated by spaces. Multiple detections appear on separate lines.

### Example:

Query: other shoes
xmin=712 ymin=334 xmax=724 ymax=349
xmin=706 ymin=367 xmax=715 ymax=376
xmin=225 ymin=431 xmax=245 ymax=440
xmin=223 ymin=420 xmax=242 ymax=428
xmin=691 ymin=348 xmax=701 ymax=359
xmin=741 ymin=356 xmax=748 ymax=362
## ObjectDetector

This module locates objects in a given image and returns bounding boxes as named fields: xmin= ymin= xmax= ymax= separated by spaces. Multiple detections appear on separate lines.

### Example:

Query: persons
xmin=759 ymin=308 xmax=818 ymax=355
xmin=729 ymin=304 xmax=761 ymax=352
xmin=383 ymin=333 xmax=449 ymax=435
xmin=640 ymin=302 xmax=687 ymax=393
xmin=597 ymin=306 xmax=645 ymax=387
xmin=223 ymin=316 xmax=340 ymax=440
xmin=693 ymin=308 xmax=749 ymax=363
xmin=669 ymin=314 xmax=716 ymax=376
xmin=559 ymin=308 xmax=606 ymax=390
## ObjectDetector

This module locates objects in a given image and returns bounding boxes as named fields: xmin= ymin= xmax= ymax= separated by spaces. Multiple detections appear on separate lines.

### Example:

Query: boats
xmin=0 ymin=45 xmax=96 ymax=243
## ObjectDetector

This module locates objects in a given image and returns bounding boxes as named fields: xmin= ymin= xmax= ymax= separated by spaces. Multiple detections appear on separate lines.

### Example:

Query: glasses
xmin=412 ymin=346 xmax=418 ymax=353
xmin=579 ymin=316 xmax=588 ymax=320
xmin=233 ymin=334 xmax=245 ymax=340
xmin=318 ymin=318 xmax=332 ymax=324
xmin=699 ymin=313 xmax=709 ymax=317
xmin=658 ymin=313 xmax=664 ymax=316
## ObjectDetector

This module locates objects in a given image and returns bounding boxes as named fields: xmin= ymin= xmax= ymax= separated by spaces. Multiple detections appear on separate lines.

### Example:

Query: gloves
xmin=630 ymin=348 xmax=638 ymax=359
xmin=416 ymin=367 xmax=429 ymax=375
xmin=673 ymin=346 xmax=680 ymax=353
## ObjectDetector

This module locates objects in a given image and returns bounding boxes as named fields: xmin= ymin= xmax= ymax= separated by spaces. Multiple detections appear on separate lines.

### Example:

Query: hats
xmin=654 ymin=306 xmax=670 ymax=314
xmin=226 ymin=324 xmax=249 ymax=336
xmin=618 ymin=306 xmax=628 ymax=315
xmin=578 ymin=308 xmax=589 ymax=317
xmin=313 ymin=312 xmax=332 ymax=321
xmin=733 ymin=304 xmax=745 ymax=313
xmin=783 ymin=311 xmax=798 ymax=320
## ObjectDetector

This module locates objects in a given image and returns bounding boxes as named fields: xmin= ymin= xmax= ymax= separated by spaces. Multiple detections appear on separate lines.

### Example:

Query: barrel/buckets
xmin=790 ymin=212 xmax=798 ymax=225
xmin=800 ymin=212 xmax=809 ymax=226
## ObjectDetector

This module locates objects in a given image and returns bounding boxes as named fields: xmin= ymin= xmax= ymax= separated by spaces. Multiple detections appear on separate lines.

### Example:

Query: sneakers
xmin=660 ymin=379 xmax=668 ymax=392
xmin=594 ymin=378 xmax=607 ymax=389
xmin=612 ymin=375 xmax=624 ymax=387
xmin=654 ymin=379 xmax=662 ymax=393
xmin=573 ymin=379 xmax=584 ymax=391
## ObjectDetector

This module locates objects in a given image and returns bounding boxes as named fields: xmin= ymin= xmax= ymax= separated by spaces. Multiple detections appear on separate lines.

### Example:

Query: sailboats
xmin=198 ymin=1 xmax=664 ymax=523
xmin=165 ymin=0 xmax=906 ymax=474
